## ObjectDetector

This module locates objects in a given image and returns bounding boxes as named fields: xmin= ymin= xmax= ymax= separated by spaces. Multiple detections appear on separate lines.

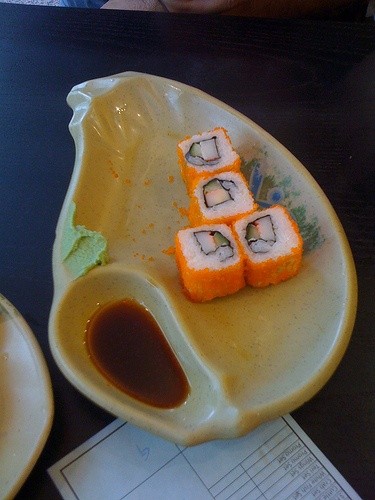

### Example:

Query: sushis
xmin=171 ymin=125 xmax=305 ymax=305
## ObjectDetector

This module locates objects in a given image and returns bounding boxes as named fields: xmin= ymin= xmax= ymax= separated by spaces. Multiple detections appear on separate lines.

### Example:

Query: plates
xmin=48 ymin=70 xmax=357 ymax=445
xmin=0 ymin=292 xmax=55 ymax=500
